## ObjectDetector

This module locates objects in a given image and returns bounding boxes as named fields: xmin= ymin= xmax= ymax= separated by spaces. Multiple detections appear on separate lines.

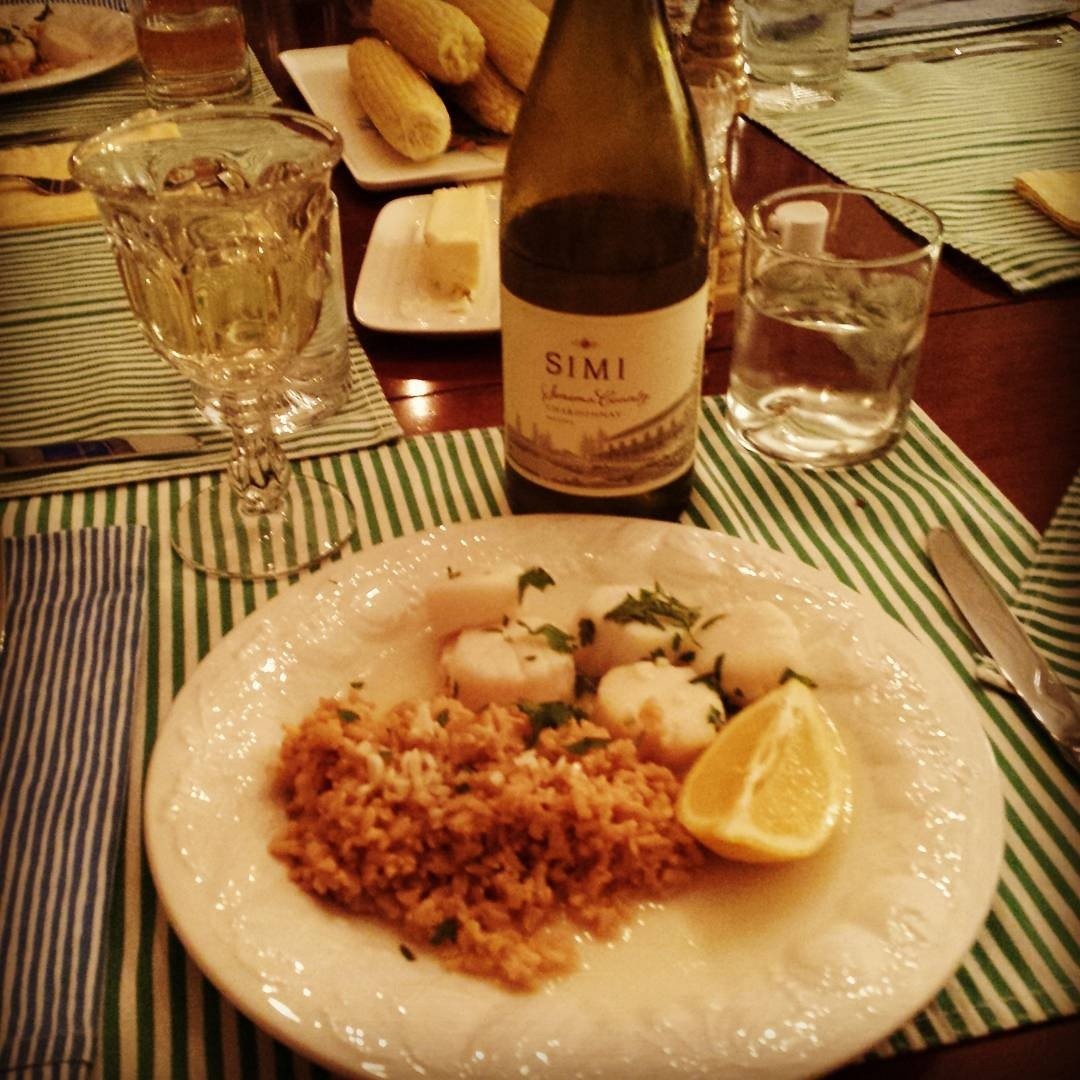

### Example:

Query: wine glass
xmin=67 ymin=105 xmax=358 ymax=579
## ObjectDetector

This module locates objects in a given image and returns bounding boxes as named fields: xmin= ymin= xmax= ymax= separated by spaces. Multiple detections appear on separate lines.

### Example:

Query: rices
xmin=270 ymin=690 xmax=709 ymax=984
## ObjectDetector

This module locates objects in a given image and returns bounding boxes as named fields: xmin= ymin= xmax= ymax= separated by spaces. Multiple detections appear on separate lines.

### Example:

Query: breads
xmin=423 ymin=182 xmax=486 ymax=295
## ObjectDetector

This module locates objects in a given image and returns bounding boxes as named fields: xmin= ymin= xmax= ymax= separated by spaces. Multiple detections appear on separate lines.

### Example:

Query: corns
xmin=348 ymin=0 xmax=553 ymax=161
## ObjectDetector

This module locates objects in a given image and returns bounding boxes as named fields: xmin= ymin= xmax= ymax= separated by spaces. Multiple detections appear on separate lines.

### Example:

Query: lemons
xmin=673 ymin=681 xmax=847 ymax=864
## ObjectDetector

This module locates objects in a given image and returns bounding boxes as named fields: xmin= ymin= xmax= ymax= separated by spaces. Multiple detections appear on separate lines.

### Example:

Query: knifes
xmin=0 ymin=127 xmax=68 ymax=149
xmin=848 ymin=35 xmax=1064 ymax=70
xmin=930 ymin=527 xmax=1080 ymax=750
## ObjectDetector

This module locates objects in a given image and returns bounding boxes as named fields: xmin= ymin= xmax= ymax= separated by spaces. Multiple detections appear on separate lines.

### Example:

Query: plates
xmin=353 ymin=191 xmax=503 ymax=333
xmin=0 ymin=2 xmax=136 ymax=94
xmin=280 ymin=44 xmax=509 ymax=190
xmin=144 ymin=514 xmax=1006 ymax=1080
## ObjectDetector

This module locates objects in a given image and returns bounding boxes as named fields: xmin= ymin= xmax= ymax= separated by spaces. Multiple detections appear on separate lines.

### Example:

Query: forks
xmin=0 ymin=174 xmax=84 ymax=196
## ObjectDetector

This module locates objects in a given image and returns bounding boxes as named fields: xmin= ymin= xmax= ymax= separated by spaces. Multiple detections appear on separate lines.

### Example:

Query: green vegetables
xmin=498 ymin=566 xmax=820 ymax=751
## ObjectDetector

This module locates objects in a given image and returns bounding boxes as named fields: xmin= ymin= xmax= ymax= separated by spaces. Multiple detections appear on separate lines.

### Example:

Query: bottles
xmin=499 ymin=0 xmax=714 ymax=521
xmin=679 ymin=0 xmax=750 ymax=185
xmin=678 ymin=60 xmax=746 ymax=349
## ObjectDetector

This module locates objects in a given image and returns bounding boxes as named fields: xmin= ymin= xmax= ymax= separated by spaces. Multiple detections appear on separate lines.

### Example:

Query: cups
xmin=726 ymin=186 xmax=945 ymax=465
xmin=743 ymin=0 xmax=853 ymax=115
xmin=131 ymin=0 xmax=251 ymax=110
